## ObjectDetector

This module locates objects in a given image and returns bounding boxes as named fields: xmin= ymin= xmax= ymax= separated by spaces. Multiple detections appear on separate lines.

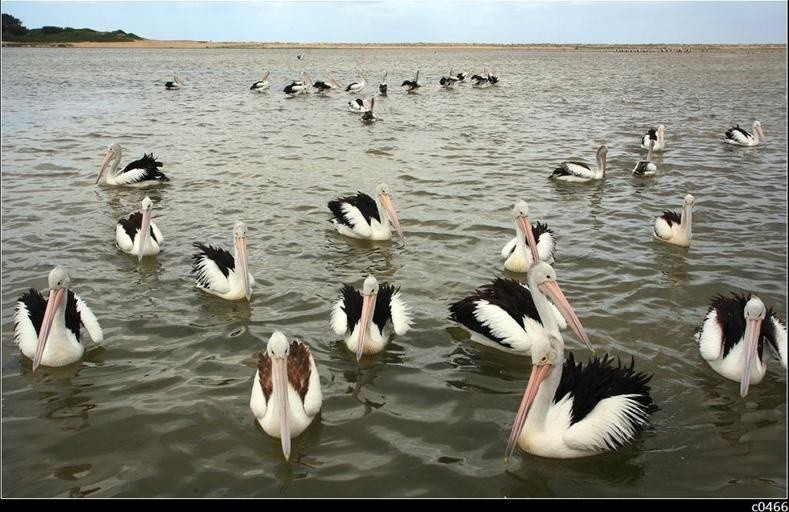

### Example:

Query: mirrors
xmin=651 ymin=193 xmax=696 ymax=247
xmin=719 ymin=120 xmax=767 ymax=146
xmin=249 ymin=327 xmax=323 ymax=463
xmin=325 ymin=182 xmax=404 ymax=241
xmin=11 ymin=264 xmax=104 ymax=375
xmin=164 ymin=75 xmax=185 ymax=90
xmin=113 ymin=194 xmax=165 ymax=263
xmin=691 ymin=290 xmax=788 ymax=399
xmin=94 ymin=143 xmax=170 ymax=189
xmin=547 ymin=122 xmax=667 ymax=183
xmin=249 ymin=67 xmax=500 ymax=98
xmin=347 ymin=97 xmax=378 ymax=123
xmin=329 ymin=271 xmax=418 ymax=364
xmin=189 ymin=219 xmax=256 ymax=303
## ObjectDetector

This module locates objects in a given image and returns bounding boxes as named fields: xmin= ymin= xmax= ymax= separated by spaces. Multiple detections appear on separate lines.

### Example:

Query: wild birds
xmin=249 ymin=67 xmax=500 ymax=98
xmin=189 ymin=219 xmax=256 ymax=303
xmin=113 ymin=194 xmax=165 ymax=263
xmin=11 ymin=264 xmax=104 ymax=375
xmin=249 ymin=327 xmax=323 ymax=463
xmin=94 ymin=143 xmax=170 ymax=189
xmin=691 ymin=290 xmax=788 ymax=399
xmin=547 ymin=122 xmax=667 ymax=183
xmin=329 ymin=271 xmax=418 ymax=364
xmin=719 ymin=120 xmax=767 ymax=146
xmin=325 ymin=182 xmax=404 ymax=241
xmin=164 ymin=75 xmax=185 ymax=90
xmin=651 ymin=193 xmax=696 ymax=247
xmin=447 ymin=199 xmax=661 ymax=469
